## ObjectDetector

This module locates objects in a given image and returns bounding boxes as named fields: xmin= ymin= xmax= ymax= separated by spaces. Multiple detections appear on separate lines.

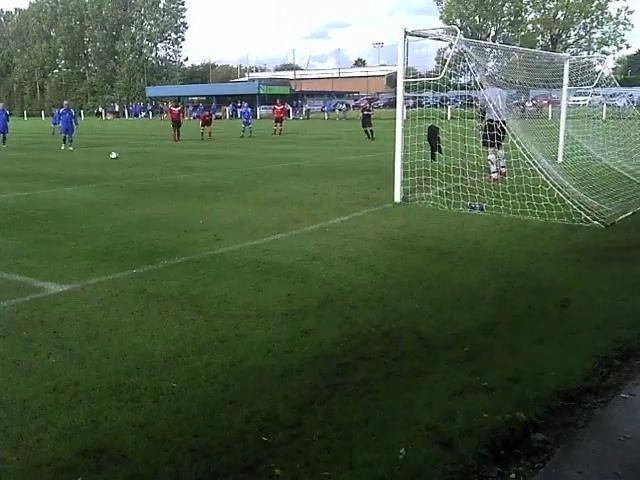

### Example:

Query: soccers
xmin=109 ymin=152 xmax=119 ymax=159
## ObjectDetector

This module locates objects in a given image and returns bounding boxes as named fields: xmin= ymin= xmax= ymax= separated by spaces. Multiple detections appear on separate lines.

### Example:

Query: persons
xmin=359 ymin=99 xmax=375 ymax=140
xmin=480 ymin=75 xmax=508 ymax=181
xmin=199 ymin=108 xmax=213 ymax=140
xmin=241 ymin=102 xmax=252 ymax=138
xmin=324 ymin=101 xmax=347 ymax=112
xmin=0 ymin=103 xmax=10 ymax=146
xmin=271 ymin=99 xmax=287 ymax=134
xmin=168 ymin=102 xmax=183 ymax=142
xmin=95 ymin=99 xmax=247 ymax=119
xmin=294 ymin=100 xmax=309 ymax=119
xmin=59 ymin=100 xmax=79 ymax=150
xmin=50 ymin=105 xmax=62 ymax=135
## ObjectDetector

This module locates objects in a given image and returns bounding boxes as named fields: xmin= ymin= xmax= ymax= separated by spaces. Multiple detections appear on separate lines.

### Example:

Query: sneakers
xmin=61 ymin=144 xmax=65 ymax=150
xmin=484 ymin=176 xmax=500 ymax=182
xmin=500 ymin=171 xmax=507 ymax=177
xmin=68 ymin=146 xmax=74 ymax=150
xmin=2 ymin=143 xmax=7 ymax=147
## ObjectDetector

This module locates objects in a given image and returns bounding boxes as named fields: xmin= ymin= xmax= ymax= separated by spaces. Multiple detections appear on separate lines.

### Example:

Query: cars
xmin=319 ymin=100 xmax=352 ymax=113
xmin=351 ymin=96 xmax=379 ymax=111
xmin=371 ymin=93 xmax=397 ymax=109
xmin=397 ymin=83 xmax=640 ymax=110
